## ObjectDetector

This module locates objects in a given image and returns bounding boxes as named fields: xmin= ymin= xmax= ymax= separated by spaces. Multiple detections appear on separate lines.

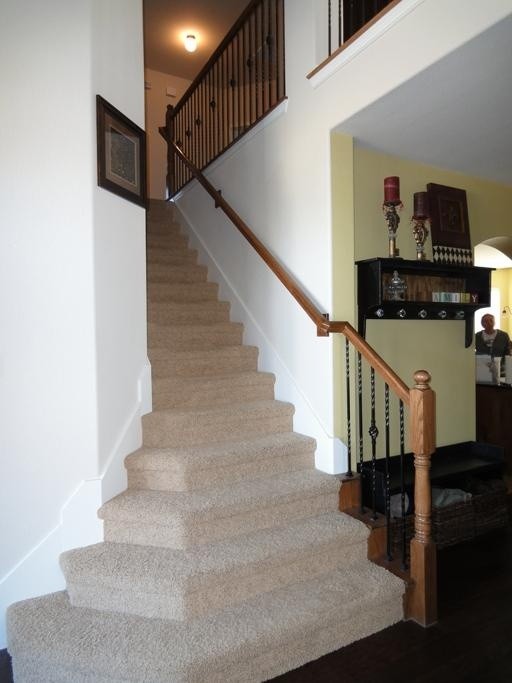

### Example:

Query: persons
xmin=475 ymin=313 xmax=512 ymax=377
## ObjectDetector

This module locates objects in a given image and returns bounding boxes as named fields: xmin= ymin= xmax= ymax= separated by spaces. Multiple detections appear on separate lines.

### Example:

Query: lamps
xmin=185 ymin=34 xmax=198 ymax=51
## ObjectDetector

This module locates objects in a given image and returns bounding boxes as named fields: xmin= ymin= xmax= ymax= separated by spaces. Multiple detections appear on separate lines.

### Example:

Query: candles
xmin=382 ymin=175 xmax=432 ymax=217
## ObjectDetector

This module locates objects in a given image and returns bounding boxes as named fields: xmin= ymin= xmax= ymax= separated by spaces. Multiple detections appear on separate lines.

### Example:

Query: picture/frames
xmin=95 ymin=94 xmax=149 ymax=211
